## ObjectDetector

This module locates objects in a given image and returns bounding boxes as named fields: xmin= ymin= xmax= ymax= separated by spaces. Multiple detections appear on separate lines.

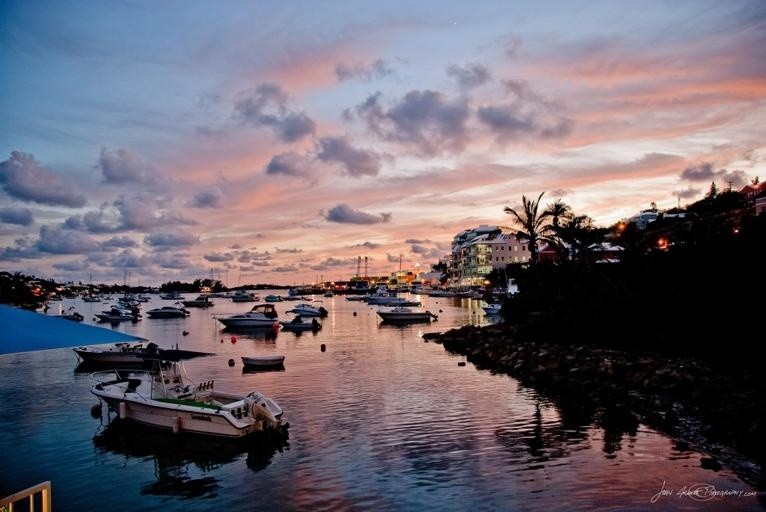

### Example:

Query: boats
xmin=72 ymin=342 xmax=166 ymax=365
xmin=91 ymin=410 xmax=290 ymax=501
xmin=241 ymin=365 xmax=286 ymax=375
xmin=89 ymin=343 xmax=284 ymax=440
xmin=240 ymin=356 xmax=285 ymax=366
xmin=55 ymin=280 xmax=507 ymax=332
xmin=73 ymin=360 xmax=171 ymax=378
